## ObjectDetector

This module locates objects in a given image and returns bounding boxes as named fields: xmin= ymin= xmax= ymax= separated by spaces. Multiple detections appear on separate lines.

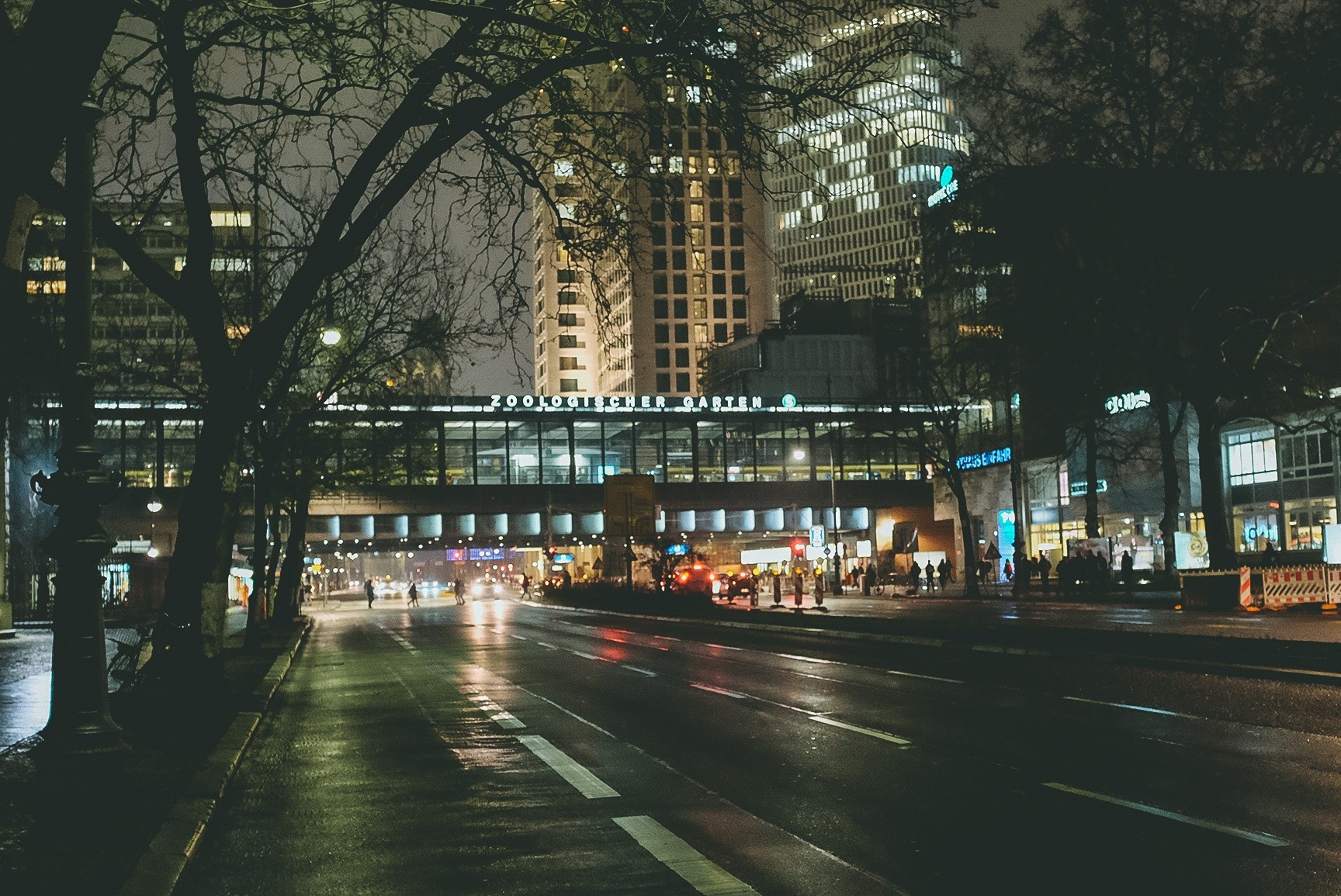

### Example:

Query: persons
xmin=563 ymin=569 xmax=572 ymax=587
xmin=978 ymin=559 xmax=993 ymax=586
xmin=366 ymin=579 xmax=375 ymax=609
xmin=241 ymin=583 xmax=249 ymax=607
xmin=1262 ymin=542 xmax=1281 ymax=567
xmin=1038 ymin=550 xmax=1136 ymax=596
xmin=519 ymin=571 xmax=532 ymax=599
xmin=944 ymin=557 xmax=956 ymax=584
xmin=1004 ymin=559 xmax=1012 ymax=582
xmin=453 ymin=579 xmax=466 ymax=605
xmin=937 ymin=559 xmax=948 ymax=590
xmin=849 ymin=562 xmax=877 ymax=590
xmin=925 ymin=560 xmax=936 ymax=593
xmin=910 ymin=561 xmax=922 ymax=590
xmin=304 ymin=580 xmax=313 ymax=606
xmin=407 ymin=582 xmax=420 ymax=607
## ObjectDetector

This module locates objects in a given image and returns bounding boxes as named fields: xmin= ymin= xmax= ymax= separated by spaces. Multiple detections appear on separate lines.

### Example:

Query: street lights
xmin=789 ymin=436 xmax=843 ymax=596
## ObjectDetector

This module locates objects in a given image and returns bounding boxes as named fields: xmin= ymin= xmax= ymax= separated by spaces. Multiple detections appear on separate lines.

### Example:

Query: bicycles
xmin=105 ymin=598 xmax=159 ymax=693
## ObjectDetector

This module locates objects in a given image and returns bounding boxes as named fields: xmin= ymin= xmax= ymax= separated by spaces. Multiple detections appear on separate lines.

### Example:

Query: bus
xmin=679 ymin=565 xmax=714 ymax=604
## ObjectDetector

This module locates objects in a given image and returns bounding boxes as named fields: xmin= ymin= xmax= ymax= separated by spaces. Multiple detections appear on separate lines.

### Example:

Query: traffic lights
xmin=546 ymin=546 xmax=555 ymax=560
xmin=795 ymin=543 xmax=804 ymax=558
